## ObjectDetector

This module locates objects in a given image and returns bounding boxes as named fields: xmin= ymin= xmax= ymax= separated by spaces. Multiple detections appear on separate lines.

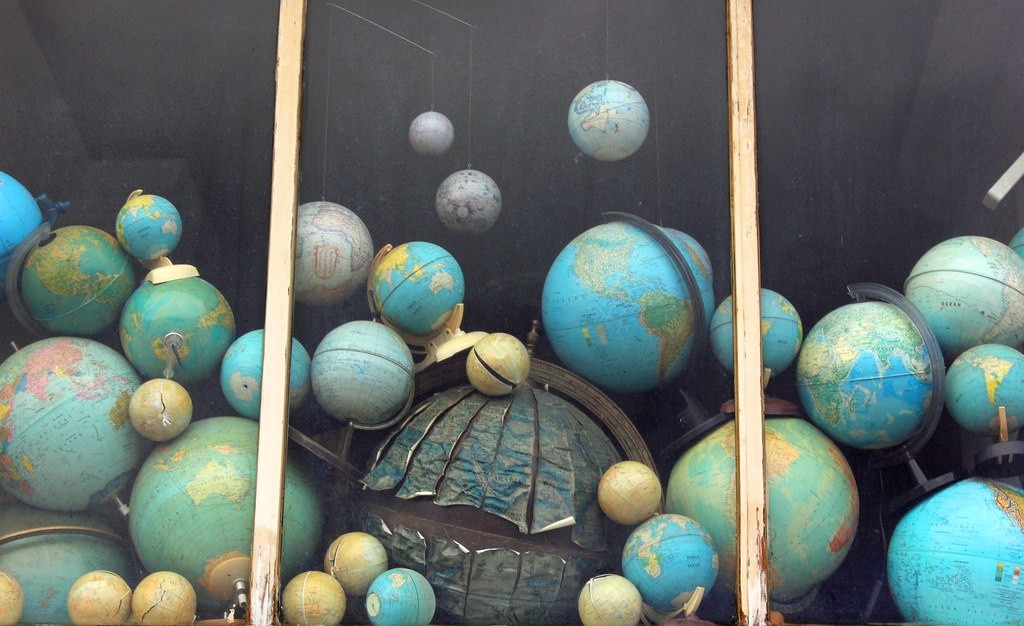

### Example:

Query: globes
xmin=409 ymin=110 xmax=454 ymax=158
xmin=567 ymin=77 xmax=651 ymax=165
xmin=0 ymin=170 xmax=1024 ymax=625
